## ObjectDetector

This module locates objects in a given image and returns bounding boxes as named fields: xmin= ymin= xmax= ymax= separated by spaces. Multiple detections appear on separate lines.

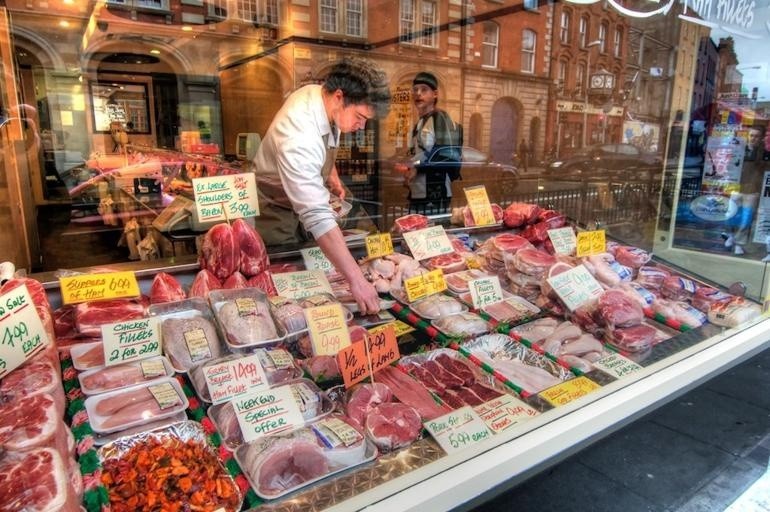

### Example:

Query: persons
xmin=251 ymin=56 xmax=392 ymax=315
xmin=517 ymin=140 xmax=530 ymax=173
xmin=127 ymin=122 xmax=137 ymax=133
xmin=724 ymin=126 xmax=770 ymax=255
xmin=395 ymin=73 xmax=463 ymax=230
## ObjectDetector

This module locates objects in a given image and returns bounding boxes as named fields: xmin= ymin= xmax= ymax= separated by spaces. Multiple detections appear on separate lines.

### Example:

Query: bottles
xmin=335 ymin=157 xmax=375 ymax=176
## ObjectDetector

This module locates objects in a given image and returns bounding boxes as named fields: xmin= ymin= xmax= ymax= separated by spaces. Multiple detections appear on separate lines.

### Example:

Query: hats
xmin=413 ymin=72 xmax=438 ymax=90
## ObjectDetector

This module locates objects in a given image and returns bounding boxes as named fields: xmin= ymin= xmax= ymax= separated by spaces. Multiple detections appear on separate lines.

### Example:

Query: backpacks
xmin=412 ymin=109 xmax=463 ymax=182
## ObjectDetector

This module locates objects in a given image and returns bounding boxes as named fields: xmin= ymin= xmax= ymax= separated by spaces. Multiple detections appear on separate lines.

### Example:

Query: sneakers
xmin=725 ymin=236 xmax=734 ymax=247
xmin=734 ymin=245 xmax=744 ymax=255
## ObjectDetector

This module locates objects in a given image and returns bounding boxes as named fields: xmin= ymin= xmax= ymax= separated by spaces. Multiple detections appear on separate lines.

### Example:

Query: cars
xmin=372 ymin=143 xmax=521 ymax=210
xmin=546 ymin=144 xmax=663 ymax=182
